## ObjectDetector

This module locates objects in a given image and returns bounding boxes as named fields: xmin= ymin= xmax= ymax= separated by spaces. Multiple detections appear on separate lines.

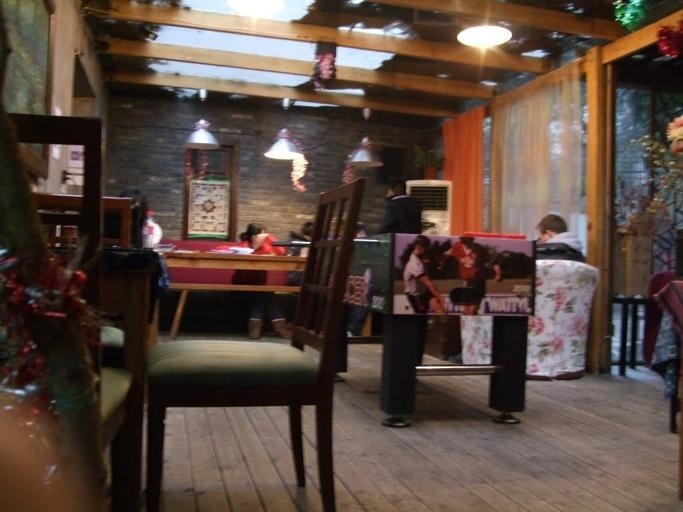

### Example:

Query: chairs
xmin=5 ymin=113 xmax=134 ymax=512
xmin=460 ymin=259 xmax=601 ymax=380
xmin=146 ymin=178 xmax=366 ymax=512
xmin=648 ymin=274 xmax=683 ymax=431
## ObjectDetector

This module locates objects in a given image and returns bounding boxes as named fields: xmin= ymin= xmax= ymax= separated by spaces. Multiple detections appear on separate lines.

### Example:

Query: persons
xmin=114 ymin=189 xmax=169 ymax=350
xmin=400 ymin=236 xmax=448 ymax=314
xmin=344 ymin=223 xmax=371 ymax=337
xmin=434 ymin=236 xmax=504 ymax=313
xmin=284 ymin=222 xmax=319 ymax=331
xmin=534 ymin=215 xmax=584 ymax=263
xmin=229 ymin=223 xmax=296 ymax=340
xmin=375 ymin=177 xmax=420 ymax=234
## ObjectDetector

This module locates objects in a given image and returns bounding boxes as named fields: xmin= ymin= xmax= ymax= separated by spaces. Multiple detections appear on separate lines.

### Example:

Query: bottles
xmin=139 ymin=207 xmax=158 ymax=249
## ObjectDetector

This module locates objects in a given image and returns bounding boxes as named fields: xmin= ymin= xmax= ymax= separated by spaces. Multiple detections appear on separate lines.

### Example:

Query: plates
xmin=171 ymin=249 xmax=202 ymax=255
xmin=153 ymin=243 xmax=176 ymax=253
xmin=229 ymin=246 xmax=256 ymax=255
xmin=204 ymin=249 xmax=236 ymax=256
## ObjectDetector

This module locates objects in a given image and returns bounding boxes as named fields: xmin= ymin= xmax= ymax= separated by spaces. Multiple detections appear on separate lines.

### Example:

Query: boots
xmin=270 ymin=318 xmax=294 ymax=340
xmin=248 ymin=317 xmax=263 ymax=339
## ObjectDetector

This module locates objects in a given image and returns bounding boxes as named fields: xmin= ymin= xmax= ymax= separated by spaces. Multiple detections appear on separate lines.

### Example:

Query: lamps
xmin=348 ymin=107 xmax=385 ymax=169
xmin=455 ymin=17 xmax=512 ymax=52
xmin=185 ymin=88 xmax=221 ymax=151
xmin=264 ymin=98 xmax=299 ymax=161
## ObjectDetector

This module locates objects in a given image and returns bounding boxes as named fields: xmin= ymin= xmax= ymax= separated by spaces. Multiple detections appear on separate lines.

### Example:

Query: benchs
xmin=165 ymin=281 xmax=301 ymax=339
